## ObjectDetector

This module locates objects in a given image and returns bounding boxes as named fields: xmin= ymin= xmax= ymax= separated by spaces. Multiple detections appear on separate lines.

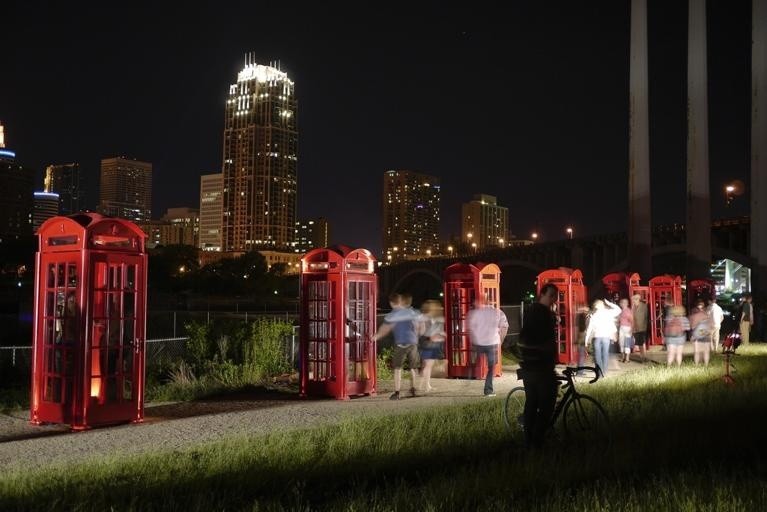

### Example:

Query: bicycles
xmin=504 ymin=366 xmax=613 ymax=456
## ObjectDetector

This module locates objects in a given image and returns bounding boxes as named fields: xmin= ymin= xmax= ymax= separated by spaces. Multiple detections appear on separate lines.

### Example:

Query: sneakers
xmin=389 ymin=393 xmax=399 ymax=400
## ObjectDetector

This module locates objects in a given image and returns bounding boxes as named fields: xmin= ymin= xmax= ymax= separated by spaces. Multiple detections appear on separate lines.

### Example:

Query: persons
xmin=465 ymin=292 xmax=509 ymax=396
xmin=56 ymin=293 xmax=84 ymax=400
xmin=518 ymin=285 xmax=557 ymax=453
xmin=369 ymin=290 xmax=448 ymax=399
xmin=585 ymin=284 xmax=754 ymax=378
